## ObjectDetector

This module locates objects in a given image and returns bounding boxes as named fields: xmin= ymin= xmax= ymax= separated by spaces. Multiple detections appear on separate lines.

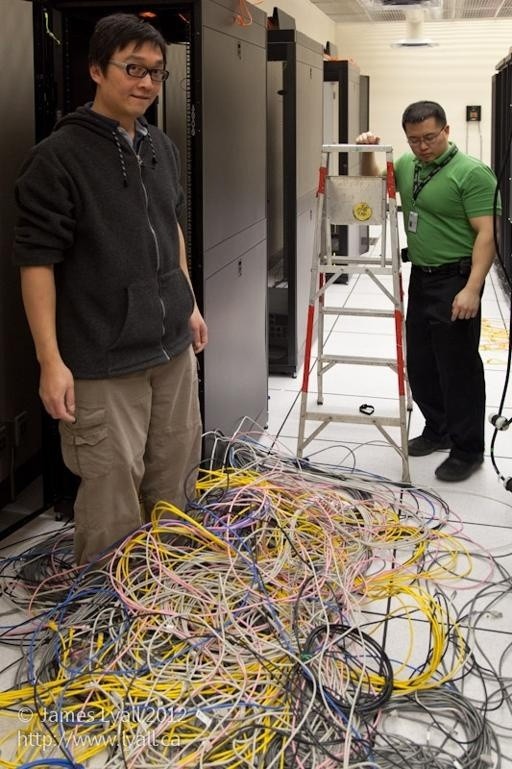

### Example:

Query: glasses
xmin=407 ymin=125 xmax=447 ymax=148
xmin=110 ymin=59 xmax=170 ymax=82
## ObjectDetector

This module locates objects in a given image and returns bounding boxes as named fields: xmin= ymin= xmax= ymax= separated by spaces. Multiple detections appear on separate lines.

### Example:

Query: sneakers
xmin=435 ymin=453 xmax=484 ymax=482
xmin=408 ymin=430 xmax=451 ymax=457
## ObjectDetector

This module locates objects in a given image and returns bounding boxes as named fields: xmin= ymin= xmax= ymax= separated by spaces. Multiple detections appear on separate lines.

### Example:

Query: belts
xmin=412 ymin=264 xmax=458 ymax=274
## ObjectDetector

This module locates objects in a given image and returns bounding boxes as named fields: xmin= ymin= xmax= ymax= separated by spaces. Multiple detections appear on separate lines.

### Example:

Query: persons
xmin=356 ymin=100 xmax=503 ymax=481
xmin=9 ymin=12 xmax=209 ymax=576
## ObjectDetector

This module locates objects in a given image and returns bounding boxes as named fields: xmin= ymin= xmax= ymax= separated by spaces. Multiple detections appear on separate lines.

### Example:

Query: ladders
xmin=295 ymin=142 xmax=410 ymax=490
